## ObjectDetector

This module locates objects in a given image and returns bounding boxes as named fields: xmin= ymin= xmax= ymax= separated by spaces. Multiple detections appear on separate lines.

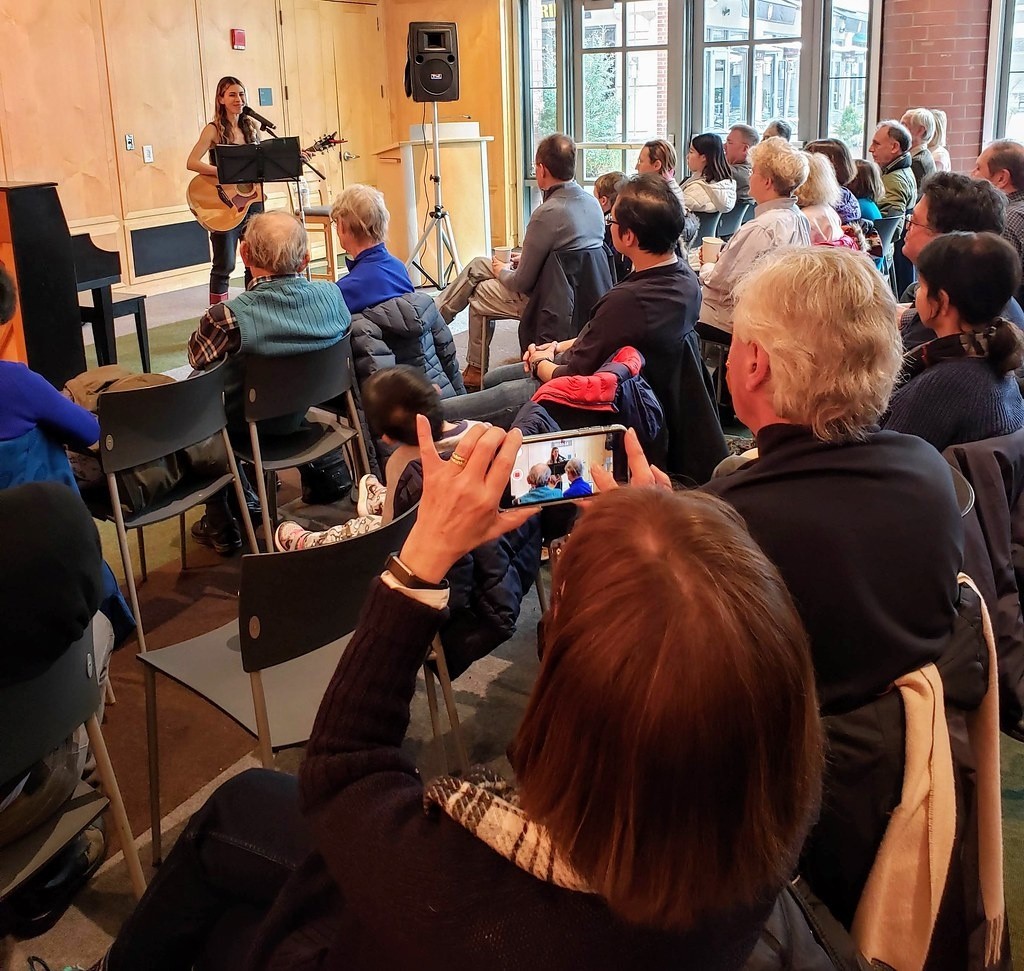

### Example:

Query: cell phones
xmin=498 ymin=423 xmax=631 ymax=512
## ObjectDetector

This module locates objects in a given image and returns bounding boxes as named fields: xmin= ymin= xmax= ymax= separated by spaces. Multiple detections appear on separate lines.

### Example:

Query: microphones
xmin=438 ymin=116 xmax=472 ymax=119
xmin=242 ymin=106 xmax=276 ymax=129
xmin=557 ymin=454 xmax=566 ymax=459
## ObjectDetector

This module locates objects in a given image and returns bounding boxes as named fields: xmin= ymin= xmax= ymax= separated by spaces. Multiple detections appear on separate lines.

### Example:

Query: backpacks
xmin=57 ymin=367 xmax=226 ymax=513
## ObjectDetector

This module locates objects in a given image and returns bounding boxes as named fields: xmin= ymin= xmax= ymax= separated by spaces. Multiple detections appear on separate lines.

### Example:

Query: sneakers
xmin=358 ymin=473 xmax=383 ymax=518
xmin=275 ymin=521 xmax=304 ymax=552
xmin=233 ymin=497 xmax=262 ymax=522
xmin=192 ymin=516 xmax=241 ymax=551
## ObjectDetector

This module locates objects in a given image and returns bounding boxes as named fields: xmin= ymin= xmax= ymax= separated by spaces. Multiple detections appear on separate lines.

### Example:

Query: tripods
xmin=405 ymin=99 xmax=463 ymax=292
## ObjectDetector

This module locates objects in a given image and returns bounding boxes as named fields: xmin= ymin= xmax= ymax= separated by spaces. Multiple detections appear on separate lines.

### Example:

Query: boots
xmin=210 ymin=293 xmax=230 ymax=305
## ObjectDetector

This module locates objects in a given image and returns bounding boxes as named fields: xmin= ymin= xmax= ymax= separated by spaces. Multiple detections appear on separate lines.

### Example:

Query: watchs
xmin=385 ymin=550 xmax=449 ymax=589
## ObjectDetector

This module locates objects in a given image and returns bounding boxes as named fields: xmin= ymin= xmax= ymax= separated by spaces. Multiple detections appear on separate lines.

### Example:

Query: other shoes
xmin=243 ymin=460 xmax=282 ymax=490
xmin=461 ymin=364 xmax=487 ymax=389
xmin=10 ymin=814 xmax=108 ymax=938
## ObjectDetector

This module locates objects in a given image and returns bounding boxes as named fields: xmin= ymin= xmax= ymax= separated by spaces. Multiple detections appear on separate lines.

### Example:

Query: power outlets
xmin=143 ymin=145 xmax=153 ymax=163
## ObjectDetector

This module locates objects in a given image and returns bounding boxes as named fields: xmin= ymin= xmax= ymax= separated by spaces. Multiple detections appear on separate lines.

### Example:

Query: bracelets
xmin=531 ymin=358 xmax=553 ymax=381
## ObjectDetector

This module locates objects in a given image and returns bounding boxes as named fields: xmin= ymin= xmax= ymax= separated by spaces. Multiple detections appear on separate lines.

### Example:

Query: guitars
xmin=186 ymin=131 xmax=348 ymax=235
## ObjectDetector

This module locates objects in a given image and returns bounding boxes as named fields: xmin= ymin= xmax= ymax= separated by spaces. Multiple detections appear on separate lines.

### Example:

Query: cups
xmin=494 ymin=246 xmax=512 ymax=264
xmin=701 ymin=237 xmax=723 ymax=263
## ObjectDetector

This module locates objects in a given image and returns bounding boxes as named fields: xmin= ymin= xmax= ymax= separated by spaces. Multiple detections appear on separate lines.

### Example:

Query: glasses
xmin=904 ymin=214 xmax=937 ymax=232
xmin=604 ymin=214 xmax=623 ymax=226
xmin=330 ymin=218 xmax=341 ymax=230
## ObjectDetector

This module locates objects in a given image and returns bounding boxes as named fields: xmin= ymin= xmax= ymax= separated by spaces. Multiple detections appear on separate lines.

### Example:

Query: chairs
xmin=288 ymin=161 xmax=353 ymax=282
xmin=0 ymin=309 xmax=1024 ymax=971
xmin=872 ymin=213 xmax=904 ymax=300
xmin=689 ymin=196 xmax=755 ymax=250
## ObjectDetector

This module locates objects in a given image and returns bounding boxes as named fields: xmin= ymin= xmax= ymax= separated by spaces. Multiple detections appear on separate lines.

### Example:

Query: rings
xmin=450 ymin=453 xmax=467 ymax=467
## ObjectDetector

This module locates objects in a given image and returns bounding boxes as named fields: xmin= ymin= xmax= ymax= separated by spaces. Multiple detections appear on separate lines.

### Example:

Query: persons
xmin=638 ymin=137 xmax=687 ymax=215
xmin=513 ymin=464 xmax=563 ymax=505
xmin=274 ymin=363 xmax=483 ymax=553
xmin=28 ymin=243 xmax=966 ymax=971
xmin=594 ymin=171 xmax=632 ymax=281
xmin=562 ymin=458 xmax=591 ymax=498
xmin=0 ymin=480 xmax=116 ymax=844
xmin=187 ymin=75 xmax=316 ymax=309
xmin=439 ymin=171 xmax=702 ymax=435
xmin=723 ymin=119 xmax=792 ymax=204
xmin=879 ymin=138 xmax=1023 ymax=453
xmin=547 ymin=447 xmax=567 ymax=489
xmin=678 ymin=133 xmax=736 ymax=213
xmin=433 ymin=132 xmax=605 ymax=393
xmin=793 ymin=138 xmax=885 ymax=270
xmin=869 ymin=108 xmax=952 ymax=242
xmin=694 ymin=135 xmax=811 ymax=343
xmin=331 ymin=183 xmax=415 ymax=314
xmin=0 ymin=258 xmax=101 ymax=447
xmin=188 ymin=209 xmax=353 ymax=557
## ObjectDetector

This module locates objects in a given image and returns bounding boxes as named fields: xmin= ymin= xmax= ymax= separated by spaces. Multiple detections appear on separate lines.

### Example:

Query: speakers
xmin=605 ymin=433 xmax=613 ymax=450
xmin=408 ymin=21 xmax=459 ymax=102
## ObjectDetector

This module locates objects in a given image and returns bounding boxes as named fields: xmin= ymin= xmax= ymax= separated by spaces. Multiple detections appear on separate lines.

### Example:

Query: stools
xmin=78 ymin=290 xmax=151 ymax=372
